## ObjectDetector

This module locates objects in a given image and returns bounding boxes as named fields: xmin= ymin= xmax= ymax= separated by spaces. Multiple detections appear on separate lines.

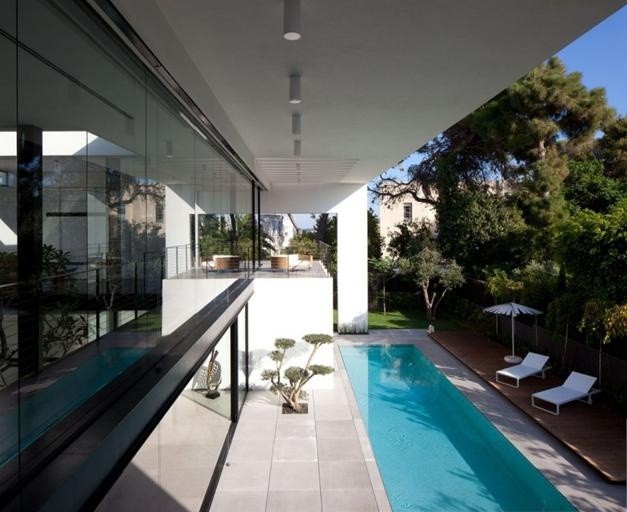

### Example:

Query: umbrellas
xmin=484 ymin=302 xmax=544 ymax=356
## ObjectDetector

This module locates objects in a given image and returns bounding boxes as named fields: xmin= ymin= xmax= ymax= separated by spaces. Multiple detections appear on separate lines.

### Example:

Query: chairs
xmin=496 ymin=352 xmax=552 ymax=389
xmin=531 ymin=371 xmax=601 ymax=415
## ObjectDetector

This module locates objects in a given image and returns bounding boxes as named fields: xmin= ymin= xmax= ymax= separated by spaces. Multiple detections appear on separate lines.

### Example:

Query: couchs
xmin=271 ymin=255 xmax=313 ymax=272
xmin=201 ymin=254 xmax=240 ymax=273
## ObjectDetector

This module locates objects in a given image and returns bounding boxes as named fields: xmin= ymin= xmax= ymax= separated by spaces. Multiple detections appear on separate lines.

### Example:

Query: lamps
xmin=283 ymin=0 xmax=301 ymax=157
